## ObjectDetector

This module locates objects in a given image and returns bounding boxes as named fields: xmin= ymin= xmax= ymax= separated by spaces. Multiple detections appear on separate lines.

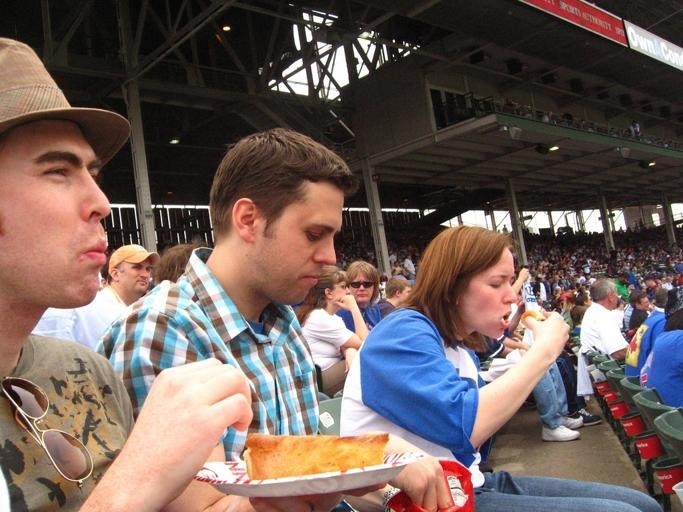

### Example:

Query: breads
xmin=243 ymin=433 xmax=389 ymax=480
xmin=521 ymin=311 xmax=547 ymax=329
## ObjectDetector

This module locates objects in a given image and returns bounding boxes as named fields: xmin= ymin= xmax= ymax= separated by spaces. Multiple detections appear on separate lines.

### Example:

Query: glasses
xmin=1 ymin=377 xmax=94 ymax=494
xmin=350 ymin=281 xmax=374 ymax=288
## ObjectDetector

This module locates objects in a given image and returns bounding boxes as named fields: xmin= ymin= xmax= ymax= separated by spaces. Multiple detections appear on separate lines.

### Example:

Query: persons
xmin=293 ymin=261 xmax=430 ymax=398
xmin=501 ymin=95 xmax=682 ymax=152
xmin=0 ymin=35 xmax=389 ymax=512
xmin=339 ymin=226 xmax=665 ymax=511
xmin=475 ymin=302 xmax=604 ymax=440
xmin=84 ymin=242 xmax=201 ymax=351
xmin=333 ymin=233 xmax=438 ymax=279
xmin=511 ymin=234 xmax=682 ymax=360
xmin=625 ymin=287 xmax=682 ymax=408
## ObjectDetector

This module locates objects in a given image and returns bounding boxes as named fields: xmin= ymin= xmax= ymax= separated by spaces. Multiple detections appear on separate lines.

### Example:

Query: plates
xmin=194 ymin=431 xmax=424 ymax=497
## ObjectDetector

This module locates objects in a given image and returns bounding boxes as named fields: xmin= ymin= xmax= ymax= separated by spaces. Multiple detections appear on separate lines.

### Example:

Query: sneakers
xmin=565 ymin=416 xmax=583 ymax=429
xmin=570 ymin=407 xmax=603 ymax=427
xmin=542 ymin=424 xmax=581 ymax=442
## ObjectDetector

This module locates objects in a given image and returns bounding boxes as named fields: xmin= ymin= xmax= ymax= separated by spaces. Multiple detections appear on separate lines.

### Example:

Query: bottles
xmin=590 ymin=368 xmax=606 ymax=382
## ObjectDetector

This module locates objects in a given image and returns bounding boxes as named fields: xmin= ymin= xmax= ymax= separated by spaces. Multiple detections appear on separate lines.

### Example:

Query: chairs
xmin=584 ymin=336 xmax=682 ymax=506
xmin=313 ymin=393 xmax=363 ymax=511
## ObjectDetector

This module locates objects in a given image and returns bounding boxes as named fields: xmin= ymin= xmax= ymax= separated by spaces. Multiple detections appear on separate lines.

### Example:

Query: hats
xmin=0 ymin=36 xmax=132 ymax=172
xmin=108 ymin=244 xmax=161 ymax=273
